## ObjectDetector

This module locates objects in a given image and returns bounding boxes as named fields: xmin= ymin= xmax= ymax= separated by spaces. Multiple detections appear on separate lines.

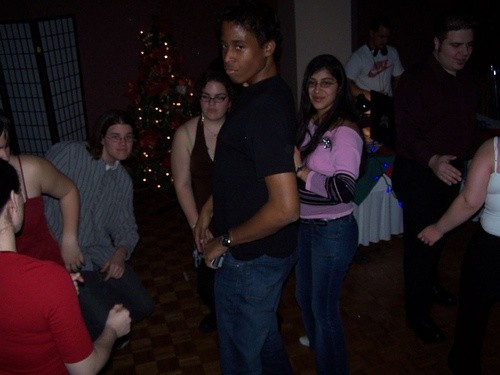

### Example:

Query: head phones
xmin=367 ymin=42 xmax=388 ymax=57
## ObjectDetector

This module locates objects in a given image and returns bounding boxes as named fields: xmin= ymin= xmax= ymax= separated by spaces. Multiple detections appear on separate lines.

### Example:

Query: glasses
xmin=105 ymin=133 xmax=136 ymax=142
xmin=200 ymin=96 xmax=228 ymax=102
xmin=308 ymin=82 xmax=336 ymax=88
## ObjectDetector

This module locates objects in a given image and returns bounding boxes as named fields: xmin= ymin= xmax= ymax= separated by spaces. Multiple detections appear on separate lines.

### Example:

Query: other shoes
xmin=115 ymin=337 xmax=130 ymax=350
xmin=300 ymin=336 xmax=310 ymax=346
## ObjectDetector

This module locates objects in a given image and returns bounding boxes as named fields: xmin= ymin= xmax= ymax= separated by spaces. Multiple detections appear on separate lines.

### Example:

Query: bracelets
xmin=296 ymin=166 xmax=304 ymax=174
xmin=300 ymin=167 xmax=310 ymax=180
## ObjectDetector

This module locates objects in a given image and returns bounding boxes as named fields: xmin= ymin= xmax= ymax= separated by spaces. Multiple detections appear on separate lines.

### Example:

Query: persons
xmin=171 ymin=3 xmax=500 ymax=375
xmin=0 ymin=109 xmax=156 ymax=375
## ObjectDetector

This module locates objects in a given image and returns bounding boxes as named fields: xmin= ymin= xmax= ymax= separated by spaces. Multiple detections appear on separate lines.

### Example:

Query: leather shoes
xmin=200 ymin=312 xmax=217 ymax=331
xmin=410 ymin=317 xmax=440 ymax=343
xmin=432 ymin=291 xmax=454 ymax=304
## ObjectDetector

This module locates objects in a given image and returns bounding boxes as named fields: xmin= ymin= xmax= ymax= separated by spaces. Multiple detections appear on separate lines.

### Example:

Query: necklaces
xmin=204 ymin=124 xmax=218 ymax=136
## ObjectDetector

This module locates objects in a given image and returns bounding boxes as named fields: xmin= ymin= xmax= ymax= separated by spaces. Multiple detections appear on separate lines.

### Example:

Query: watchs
xmin=222 ymin=231 xmax=231 ymax=248
